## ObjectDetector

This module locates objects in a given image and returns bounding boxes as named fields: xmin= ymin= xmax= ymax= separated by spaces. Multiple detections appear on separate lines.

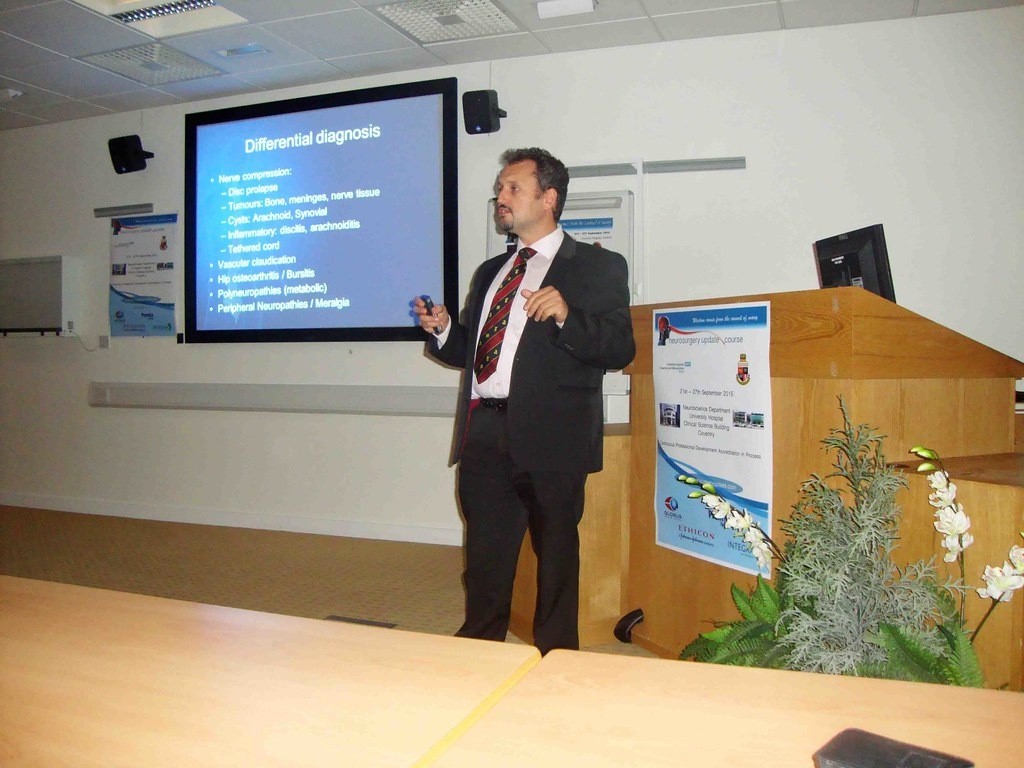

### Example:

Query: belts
xmin=479 ymin=397 xmax=508 ymax=414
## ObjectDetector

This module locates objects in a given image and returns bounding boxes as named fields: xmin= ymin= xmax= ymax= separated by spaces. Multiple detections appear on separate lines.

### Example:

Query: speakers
xmin=462 ymin=89 xmax=502 ymax=134
xmin=108 ymin=135 xmax=147 ymax=174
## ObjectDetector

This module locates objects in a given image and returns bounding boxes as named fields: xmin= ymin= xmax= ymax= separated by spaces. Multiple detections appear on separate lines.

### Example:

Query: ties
xmin=474 ymin=248 xmax=539 ymax=384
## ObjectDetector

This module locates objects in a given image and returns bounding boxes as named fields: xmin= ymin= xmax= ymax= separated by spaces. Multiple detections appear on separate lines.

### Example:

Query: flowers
xmin=678 ymin=405 xmax=1024 ymax=688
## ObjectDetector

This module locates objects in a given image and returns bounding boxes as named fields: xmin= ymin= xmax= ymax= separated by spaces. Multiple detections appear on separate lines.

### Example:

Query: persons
xmin=414 ymin=146 xmax=637 ymax=657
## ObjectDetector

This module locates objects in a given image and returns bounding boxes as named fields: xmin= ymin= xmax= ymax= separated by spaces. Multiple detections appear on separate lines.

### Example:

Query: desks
xmin=0 ymin=576 xmax=1024 ymax=768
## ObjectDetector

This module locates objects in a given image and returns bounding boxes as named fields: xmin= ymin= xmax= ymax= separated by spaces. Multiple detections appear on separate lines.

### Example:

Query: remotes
xmin=419 ymin=295 xmax=443 ymax=334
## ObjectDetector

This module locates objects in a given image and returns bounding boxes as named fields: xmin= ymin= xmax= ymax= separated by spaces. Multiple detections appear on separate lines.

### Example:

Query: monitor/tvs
xmin=815 ymin=223 xmax=897 ymax=304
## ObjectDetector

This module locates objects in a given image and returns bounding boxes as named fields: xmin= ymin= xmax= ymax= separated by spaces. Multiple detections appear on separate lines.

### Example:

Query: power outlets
xmin=99 ymin=335 xmax=110 ymax=349
